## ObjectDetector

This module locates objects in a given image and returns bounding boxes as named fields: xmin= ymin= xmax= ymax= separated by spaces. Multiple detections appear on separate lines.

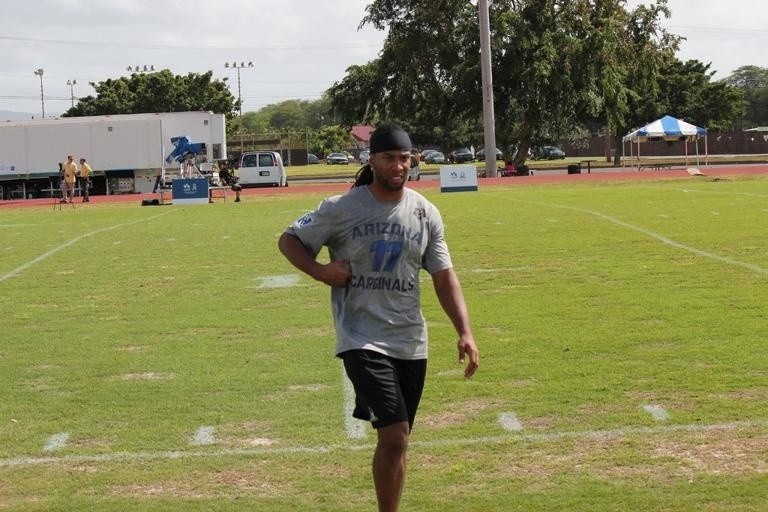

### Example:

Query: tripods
xmin=53 ymin=163 xmax=75 ymax=211
xmin=184 ymin=159 xmax=204 ymax=179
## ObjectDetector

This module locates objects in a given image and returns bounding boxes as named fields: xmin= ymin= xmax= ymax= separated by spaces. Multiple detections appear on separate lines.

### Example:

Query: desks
xmin=580 ymin=159 xmax=598 ymax=174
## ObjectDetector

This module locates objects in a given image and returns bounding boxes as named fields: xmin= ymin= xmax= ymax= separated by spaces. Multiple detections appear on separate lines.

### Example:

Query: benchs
xmin=158 ymin=189 xmax=172 ymax=205
xmin=477 ymin=167 xmax=517 ymax=177
xmin=637 ymin=162 xmax=673 ymax=172
xmin=208 ymin=185 xmax=229 ymax=203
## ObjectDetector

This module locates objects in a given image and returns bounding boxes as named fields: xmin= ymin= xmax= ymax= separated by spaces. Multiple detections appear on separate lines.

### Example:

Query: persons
xmin=79 ymin=158 xmax=94 ymax=203
xmin=276 ymin=118 xmax=478 ymax=512
xmin=499 ymin=161 xmax=516 ymax=177
xmin=221 ymin=161 xmax=241 ymax=203
xmin=58 ymin=155 xmax=78 ymax=202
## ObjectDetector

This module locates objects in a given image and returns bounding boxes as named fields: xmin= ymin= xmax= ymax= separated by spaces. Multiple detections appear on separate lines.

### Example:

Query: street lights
xmin=124 ymin=62 xmax=156 ymax=77
xmin=64 ymin=78 xmax=77 ymax=110
xmin=223 ymin=59 xmax=257 ymax=156
xmin=32 ymin=68 xmax=47 ymax=119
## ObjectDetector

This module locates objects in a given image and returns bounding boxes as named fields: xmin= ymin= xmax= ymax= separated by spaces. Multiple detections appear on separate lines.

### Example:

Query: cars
xmin=408 ymin=145 xmax=568 ymax=183
xmin=307 ymin=149 xmax=370 ymax=167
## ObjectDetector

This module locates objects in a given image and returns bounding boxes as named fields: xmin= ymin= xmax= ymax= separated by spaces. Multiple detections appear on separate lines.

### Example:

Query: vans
xmin=235 ymin=150 xmax=288 ymax=190
